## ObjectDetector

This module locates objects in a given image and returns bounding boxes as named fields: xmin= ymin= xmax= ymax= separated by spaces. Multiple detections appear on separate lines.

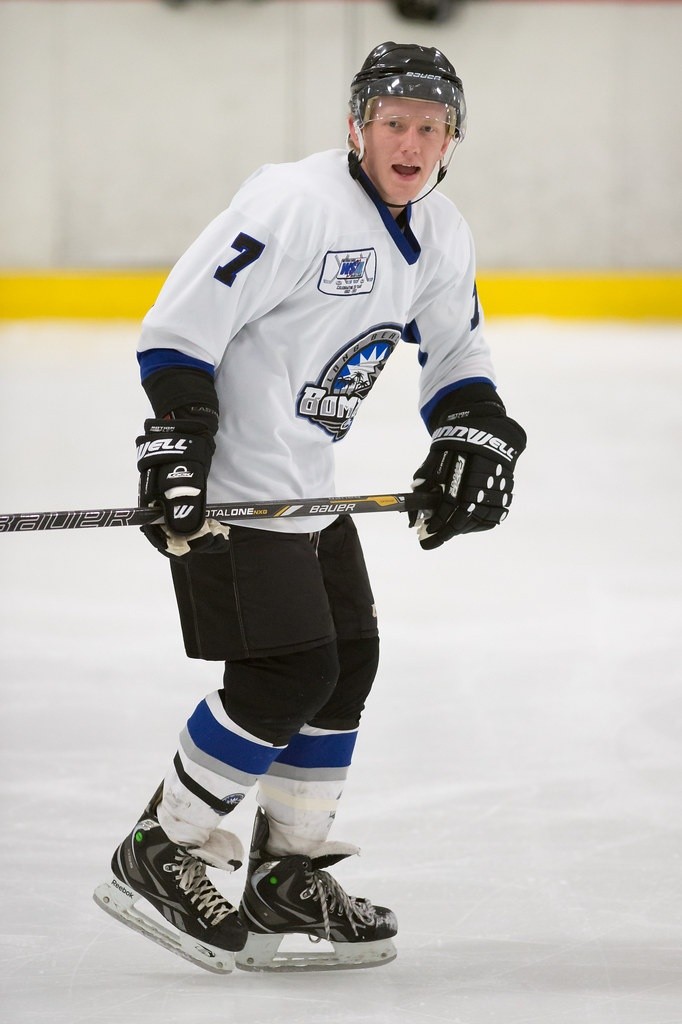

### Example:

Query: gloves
xmin=134 ymin=418 xmax=232 ymax=561
xmin=410 ymin=404 xmax=526 ymax=550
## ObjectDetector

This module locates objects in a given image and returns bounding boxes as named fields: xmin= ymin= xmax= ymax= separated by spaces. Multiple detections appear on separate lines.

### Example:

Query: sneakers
xmin=232 ymin=806 xmax=398 ymax=971
xmin=93 ymin=779 xmax=248 ymax=973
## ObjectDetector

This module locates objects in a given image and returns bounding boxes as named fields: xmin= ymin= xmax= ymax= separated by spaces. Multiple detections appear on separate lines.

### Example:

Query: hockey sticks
xmin=1 ymin=489 xmax=422 ymax=534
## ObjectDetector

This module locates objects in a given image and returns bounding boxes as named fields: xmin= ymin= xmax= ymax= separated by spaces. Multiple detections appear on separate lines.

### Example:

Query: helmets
xmin=350 ymin=42 xmax=466 ymax=140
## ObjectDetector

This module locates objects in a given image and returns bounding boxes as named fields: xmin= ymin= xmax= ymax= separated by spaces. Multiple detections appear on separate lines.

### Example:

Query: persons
xmin=94 ymin=41 xmax=527 ymax=974
